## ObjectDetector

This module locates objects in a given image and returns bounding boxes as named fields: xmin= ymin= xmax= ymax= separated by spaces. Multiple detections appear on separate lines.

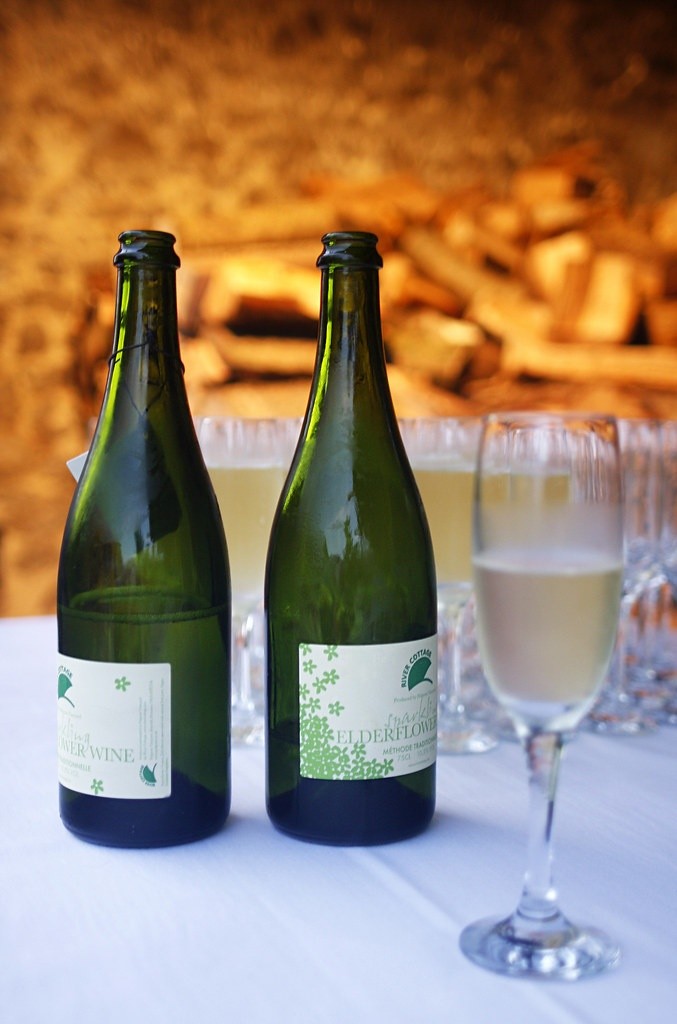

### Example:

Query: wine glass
xmin=190 ymin=412 xmax=676 ymax=982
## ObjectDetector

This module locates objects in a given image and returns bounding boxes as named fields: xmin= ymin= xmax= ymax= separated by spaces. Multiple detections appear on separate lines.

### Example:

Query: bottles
xmin=264 ymin=230 xmax=436 ymax=846
xmin=55 ymin=229 xmax=232 ymax=849
